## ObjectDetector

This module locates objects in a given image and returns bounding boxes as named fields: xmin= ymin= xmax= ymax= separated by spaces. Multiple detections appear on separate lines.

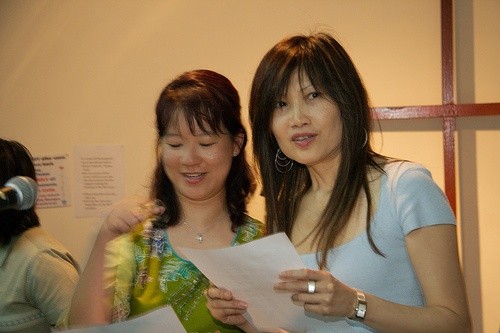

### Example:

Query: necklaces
xmin=181 ymin=212 xmax=229 ymax=242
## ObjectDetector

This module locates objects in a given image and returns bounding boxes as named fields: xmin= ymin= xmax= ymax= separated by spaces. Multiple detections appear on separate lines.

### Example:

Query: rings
xmin=307 ymin=279 xmax=316 ymax=294
xmin=205 ymin=287 xmax=211 ymax=299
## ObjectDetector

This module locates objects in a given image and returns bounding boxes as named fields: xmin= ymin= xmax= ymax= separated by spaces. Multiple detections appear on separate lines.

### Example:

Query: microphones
xmin=0 ymin=176 xmax=37 ymax=211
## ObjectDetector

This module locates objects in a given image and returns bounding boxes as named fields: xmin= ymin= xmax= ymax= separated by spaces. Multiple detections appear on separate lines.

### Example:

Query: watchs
xmin=348 ymin=288 xmax=367 ymax=322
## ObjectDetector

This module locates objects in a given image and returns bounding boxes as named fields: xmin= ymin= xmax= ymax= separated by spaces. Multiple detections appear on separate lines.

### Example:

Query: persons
xmin=204 ymin=32 xmax=472 ymax=333
xmin=0 ymin=138 xmax=82 ymax=333
xmin=70 ymin=69 xmax=267 ymax=333
